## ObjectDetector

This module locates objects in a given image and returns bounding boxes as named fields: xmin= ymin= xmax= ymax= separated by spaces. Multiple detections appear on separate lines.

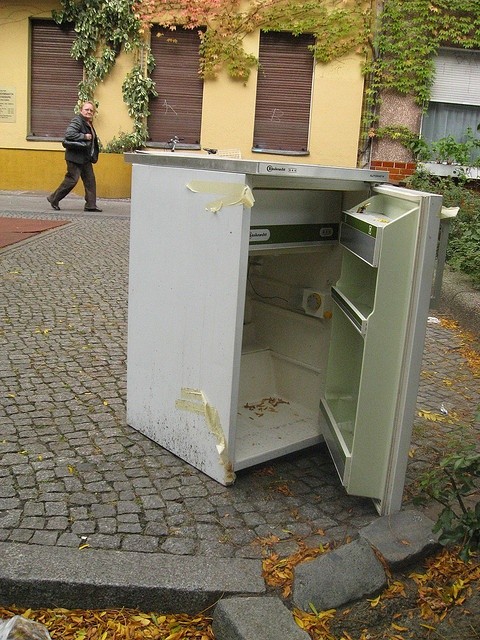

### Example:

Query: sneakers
xmin=84 ymin=207 xmax=102 ymax=212
xmin=47 ymin=197 xmax=60 ymax=210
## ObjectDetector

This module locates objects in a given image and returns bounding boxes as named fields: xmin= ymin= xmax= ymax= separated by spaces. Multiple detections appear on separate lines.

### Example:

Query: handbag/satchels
xmin=62 ymin=138 xmax=92 ymax=151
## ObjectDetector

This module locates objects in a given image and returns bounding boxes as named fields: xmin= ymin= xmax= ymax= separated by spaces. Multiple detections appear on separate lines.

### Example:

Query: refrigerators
xmin=117 ymin=147 xmax=445 ymax=518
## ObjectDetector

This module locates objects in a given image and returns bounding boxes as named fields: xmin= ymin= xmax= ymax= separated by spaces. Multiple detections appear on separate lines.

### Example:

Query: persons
xmin=46 ymin=101 xmax=102 ymax=212
